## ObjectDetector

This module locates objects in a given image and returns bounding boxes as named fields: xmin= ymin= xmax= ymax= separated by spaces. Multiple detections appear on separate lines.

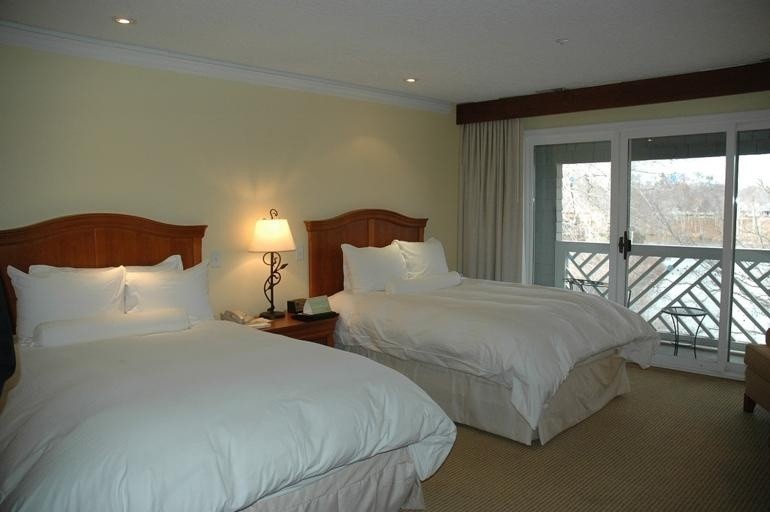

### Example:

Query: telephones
xmin=222 ymin=310 xmax=253 ymax=324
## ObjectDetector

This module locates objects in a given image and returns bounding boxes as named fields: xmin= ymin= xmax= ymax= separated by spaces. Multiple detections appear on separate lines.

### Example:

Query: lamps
xmin=248 ymin=207 xmax=297 ymax=319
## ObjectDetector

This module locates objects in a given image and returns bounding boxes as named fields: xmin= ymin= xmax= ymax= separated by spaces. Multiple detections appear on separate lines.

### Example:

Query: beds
xmin=303 ymin=208 xmax=662 ymax=448
xmin=0 ymin=212 xmax=459 ymax=512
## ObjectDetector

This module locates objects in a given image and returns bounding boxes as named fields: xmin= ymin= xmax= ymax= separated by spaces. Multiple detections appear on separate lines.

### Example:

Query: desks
xmin=563 ymin=277 xmax=609 ymax=291
xmin=662 ymin=306 xmax=708 ymax=359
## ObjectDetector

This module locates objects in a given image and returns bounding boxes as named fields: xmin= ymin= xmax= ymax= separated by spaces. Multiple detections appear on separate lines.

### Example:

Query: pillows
xmin=391 ymin=235 xmax=449 ymax=279
xmin=28 ymin=265 xmax=114 ymax=275
xmin=7 ymin=264 xmax=126 ymax=344
xmin=342 ymin=253 xmax=352 ymax=292
xmin=124 ymin=259 xmax=214 ymax=326
xmin=340 ymin=243 xmax=409 ymax=296
xmin=125 ymin=254 xmax=184 ymax=271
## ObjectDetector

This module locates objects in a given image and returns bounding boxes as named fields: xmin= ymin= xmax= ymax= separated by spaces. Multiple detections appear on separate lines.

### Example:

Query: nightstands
xmin=241 ymin=310 xmax=340 ymax=349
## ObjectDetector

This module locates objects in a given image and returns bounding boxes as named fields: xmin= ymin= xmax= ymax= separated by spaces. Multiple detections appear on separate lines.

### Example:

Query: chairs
xmin=743 ymin=326 xmax=770 ymax=413
